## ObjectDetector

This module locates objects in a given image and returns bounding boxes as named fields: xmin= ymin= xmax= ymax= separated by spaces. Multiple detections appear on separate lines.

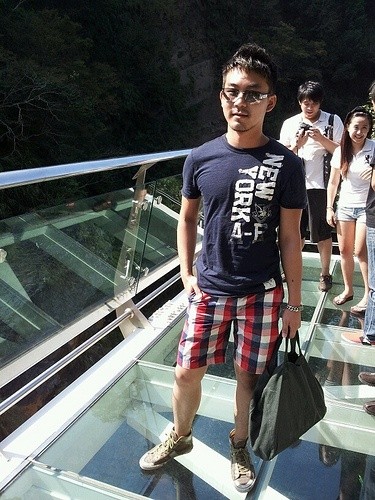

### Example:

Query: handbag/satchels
xmin=248 ymin=329 xmax=327 ymax=462
xmin=322 ymin=113 xmax=343 ymax=189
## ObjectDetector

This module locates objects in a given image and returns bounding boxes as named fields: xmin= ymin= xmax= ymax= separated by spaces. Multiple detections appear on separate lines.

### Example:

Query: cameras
xmin=299 ymin=123 xmax=312 ymax=136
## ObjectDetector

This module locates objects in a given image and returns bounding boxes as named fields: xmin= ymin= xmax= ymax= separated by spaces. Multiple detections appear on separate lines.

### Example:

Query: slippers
xmin=350 ymin=304 xmax=367 ymax=313
xmin=333 ymin=291 xmax=354 ymax=305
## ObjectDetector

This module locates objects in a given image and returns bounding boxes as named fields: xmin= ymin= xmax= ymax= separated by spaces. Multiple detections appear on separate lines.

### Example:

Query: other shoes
xmin=320 ymin=274 xmax=332 ymax=291
xmin=281 ymin=272 xmax=287 ymax=282
xmin=358 ymin=371 xmax=375 ymax=387
xmin=363 ymin=400 xmax=375 ymax=416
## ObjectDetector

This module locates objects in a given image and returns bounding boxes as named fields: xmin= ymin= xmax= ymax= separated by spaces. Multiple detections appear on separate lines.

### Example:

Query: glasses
xmin=221 ymin=87 xmax=273 ymax=104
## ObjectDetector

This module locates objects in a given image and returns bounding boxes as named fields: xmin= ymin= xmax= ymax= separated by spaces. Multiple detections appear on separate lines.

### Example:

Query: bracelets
xmin=326 ymin=207 xmax=334 ymax=210
xmin=286 ymin=304 xmax=304 ymax=312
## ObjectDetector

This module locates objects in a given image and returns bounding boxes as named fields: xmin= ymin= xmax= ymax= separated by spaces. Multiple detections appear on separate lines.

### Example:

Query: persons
xmin=139 ymin=44 xmax=306 ymax=493
xmin=278 ymin=81 xmax=375 ymax=416
xmin=140 ymin=288 xmax=375 ymax=500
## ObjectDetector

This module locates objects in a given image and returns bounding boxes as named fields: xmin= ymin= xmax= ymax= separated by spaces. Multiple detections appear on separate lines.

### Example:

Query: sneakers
xmin=340 ymin=331 xmax=375 ymax=346
xmin=139 ymin=426 xmax=193 ymax=471
xmin=228 ymin=429 xmax=257 ymax=492
